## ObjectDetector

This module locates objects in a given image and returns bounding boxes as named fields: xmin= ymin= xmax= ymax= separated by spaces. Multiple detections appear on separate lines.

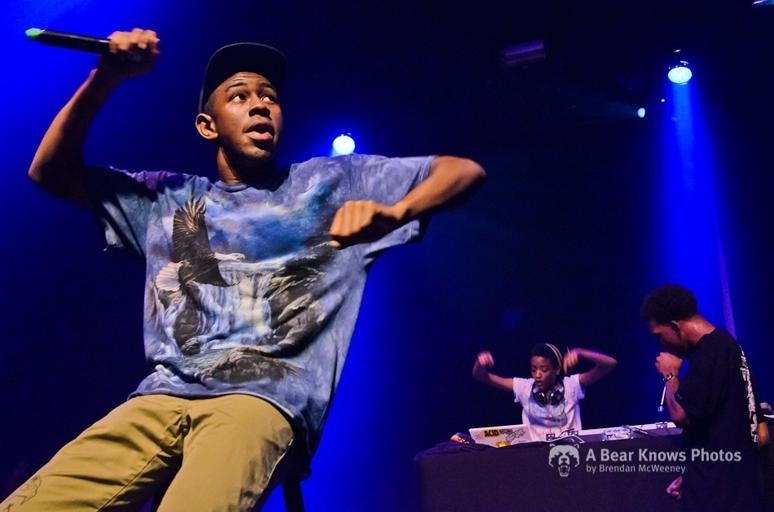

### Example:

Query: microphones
xmin=27 ymin=25 xmax=149 ymax=60
xmin=657 ymin=384 xmax=666 ymax=412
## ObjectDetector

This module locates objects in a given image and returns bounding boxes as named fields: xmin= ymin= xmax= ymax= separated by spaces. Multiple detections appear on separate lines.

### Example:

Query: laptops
xmin=469 ymin=424 xmax=533 ymax=448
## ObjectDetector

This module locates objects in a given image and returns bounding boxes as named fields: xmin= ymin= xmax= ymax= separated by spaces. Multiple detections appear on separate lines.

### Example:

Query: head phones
xmin=530 ymin=380 xmax=565 ymax=405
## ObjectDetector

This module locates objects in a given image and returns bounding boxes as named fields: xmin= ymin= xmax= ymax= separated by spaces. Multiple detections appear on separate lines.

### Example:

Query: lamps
xmin=502 ymin=39 xmax=545 ymax=68
xmin=666 ymin=41 xmax=693 ymax=84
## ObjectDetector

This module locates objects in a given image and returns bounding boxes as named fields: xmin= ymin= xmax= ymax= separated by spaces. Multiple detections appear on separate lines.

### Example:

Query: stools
xmin=151 ymin=440 xmax=312 ymax=512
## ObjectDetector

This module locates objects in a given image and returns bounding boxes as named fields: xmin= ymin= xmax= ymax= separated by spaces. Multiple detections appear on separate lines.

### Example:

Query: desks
xmin=416 ymin=422 xmax=691 ymax=512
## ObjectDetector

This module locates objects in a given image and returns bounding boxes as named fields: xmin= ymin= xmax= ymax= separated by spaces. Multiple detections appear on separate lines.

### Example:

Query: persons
xmin=0 ymin=26 xmax=485 ymax=511
xmin=641 ymin=282 xmax=772 ymax=512
xmin=473 ymin=341 xmax=618 ymax=431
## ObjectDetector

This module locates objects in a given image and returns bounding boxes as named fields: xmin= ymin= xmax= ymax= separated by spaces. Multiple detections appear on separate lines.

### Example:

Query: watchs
xmin=661 ymin=371 xmax=674 ymax=382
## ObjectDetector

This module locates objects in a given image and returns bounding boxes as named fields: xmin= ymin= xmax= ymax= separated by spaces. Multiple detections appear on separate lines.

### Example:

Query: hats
xmin=199 ymin=42 xmax=285 ymax=113
